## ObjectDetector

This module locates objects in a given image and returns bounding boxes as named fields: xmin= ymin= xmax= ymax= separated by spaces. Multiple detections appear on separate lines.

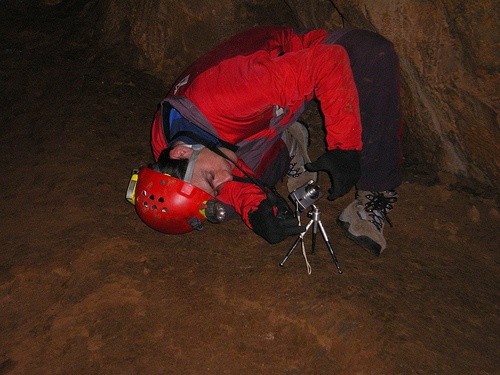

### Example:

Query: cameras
xmin=288 ymin=179 xmax=322 ymax=212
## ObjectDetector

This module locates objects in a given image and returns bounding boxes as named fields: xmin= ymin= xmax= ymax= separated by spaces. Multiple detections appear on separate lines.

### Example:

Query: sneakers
xmin=281 ymin=123 xmax=317 ymax=194
xmin=337 ymin=190 xmax=399 ymax=256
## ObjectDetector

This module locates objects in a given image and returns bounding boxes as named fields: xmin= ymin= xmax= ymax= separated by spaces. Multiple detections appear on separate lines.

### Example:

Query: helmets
xmin=126 ymin=166 xmax=226 ymax=235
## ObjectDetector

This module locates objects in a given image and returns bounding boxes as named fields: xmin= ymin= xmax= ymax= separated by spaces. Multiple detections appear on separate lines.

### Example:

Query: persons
xmin=134 ymin=25 xmax=399 ymax=255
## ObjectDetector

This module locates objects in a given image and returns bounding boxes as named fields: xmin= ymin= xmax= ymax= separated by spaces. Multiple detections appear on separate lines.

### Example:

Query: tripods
xmin=280 ymin=204 xmax=342 ymax=274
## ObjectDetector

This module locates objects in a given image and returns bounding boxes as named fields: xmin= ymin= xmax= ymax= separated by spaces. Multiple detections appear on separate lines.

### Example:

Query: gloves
xmin=304 ymin=150 xmax=361 ymax=201
xmin=248 ymin=202 xmax=305 ymax=246
